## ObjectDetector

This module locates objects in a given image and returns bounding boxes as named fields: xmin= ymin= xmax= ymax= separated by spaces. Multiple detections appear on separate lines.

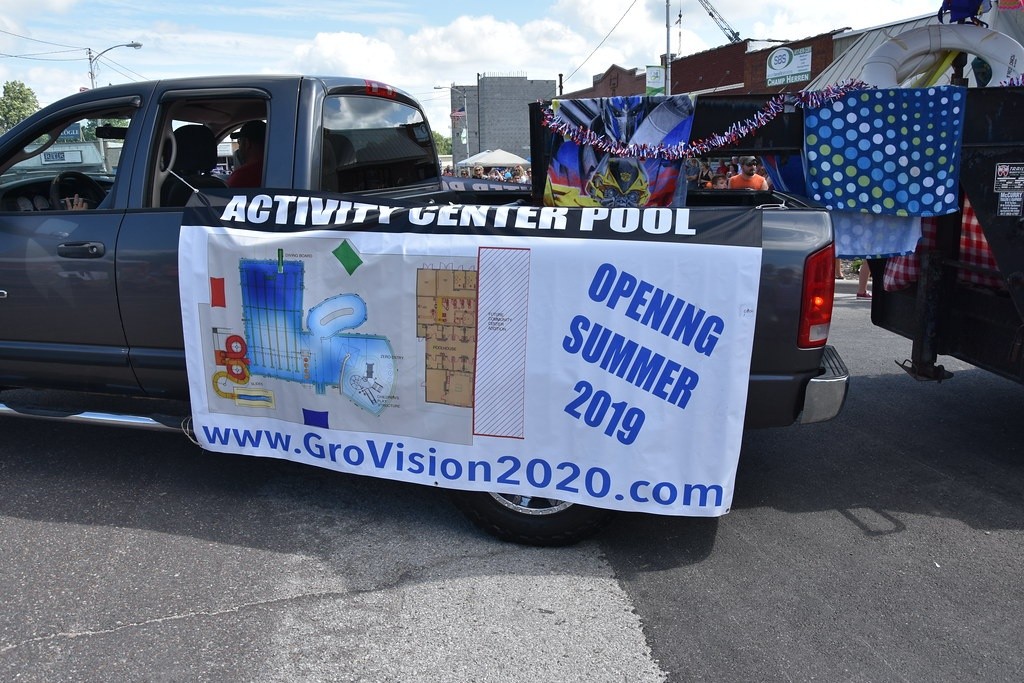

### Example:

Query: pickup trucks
xmin=1 ymin=75 xmax=852 ymax=546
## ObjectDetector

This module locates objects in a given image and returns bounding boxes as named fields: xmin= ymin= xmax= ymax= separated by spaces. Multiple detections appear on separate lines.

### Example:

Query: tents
xmin=465 ymin=149 xmax=530 ymax=177
xmin=453 ymin=149 xmax=492 ymax=178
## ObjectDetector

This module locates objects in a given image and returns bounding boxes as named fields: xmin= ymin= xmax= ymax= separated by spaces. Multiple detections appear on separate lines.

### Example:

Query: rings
xmin=73 ymin=203 xmax=78 ymax=206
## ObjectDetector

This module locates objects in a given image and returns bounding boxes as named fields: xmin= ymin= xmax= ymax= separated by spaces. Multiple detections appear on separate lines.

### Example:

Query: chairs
xmin=160 ymin=125 xmax=230 ymax=207
xmin=323 ymin=134 xmax=356 ymax=194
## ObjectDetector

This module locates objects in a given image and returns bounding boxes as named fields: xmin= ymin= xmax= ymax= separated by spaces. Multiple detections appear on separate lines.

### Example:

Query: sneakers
xmin=857 ymin=290 xmax=872 ymax=300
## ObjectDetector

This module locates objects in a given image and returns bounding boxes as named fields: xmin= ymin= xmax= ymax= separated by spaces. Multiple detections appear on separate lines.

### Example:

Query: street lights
xmin=434 ymin=86 xmax=470 ymax=159
xmin=88 ymin=42 xmax=143 ymax=88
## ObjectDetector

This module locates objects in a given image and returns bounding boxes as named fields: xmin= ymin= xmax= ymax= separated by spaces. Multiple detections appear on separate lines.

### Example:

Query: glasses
xmin=238 ymin=139 xmax=248 ymax=144
xmin=745 ymin=162 xmax=757 ymax=167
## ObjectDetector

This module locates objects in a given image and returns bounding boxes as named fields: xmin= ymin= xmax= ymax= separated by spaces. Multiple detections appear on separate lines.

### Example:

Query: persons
xmin=223 ymin=119 xmax=267 ymax=190
xmin=65 ymin=185 xmax=115 ymax=211
xmin=835 ymin=258 xmax=845 ymax=279
xmin=856 ymin=258 xmax=872 ymax=299
xmin=439 ymin=160 xmax=532 ymax=185
xmin=686 ymin=156 xmax=774 ymax=192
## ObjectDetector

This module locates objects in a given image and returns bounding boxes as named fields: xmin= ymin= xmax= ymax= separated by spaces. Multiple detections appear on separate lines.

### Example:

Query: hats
xmin=230 ymin=120 xmax=267 ymax=139
xmin=739 ymin=155 xmax=756 ymax=166
